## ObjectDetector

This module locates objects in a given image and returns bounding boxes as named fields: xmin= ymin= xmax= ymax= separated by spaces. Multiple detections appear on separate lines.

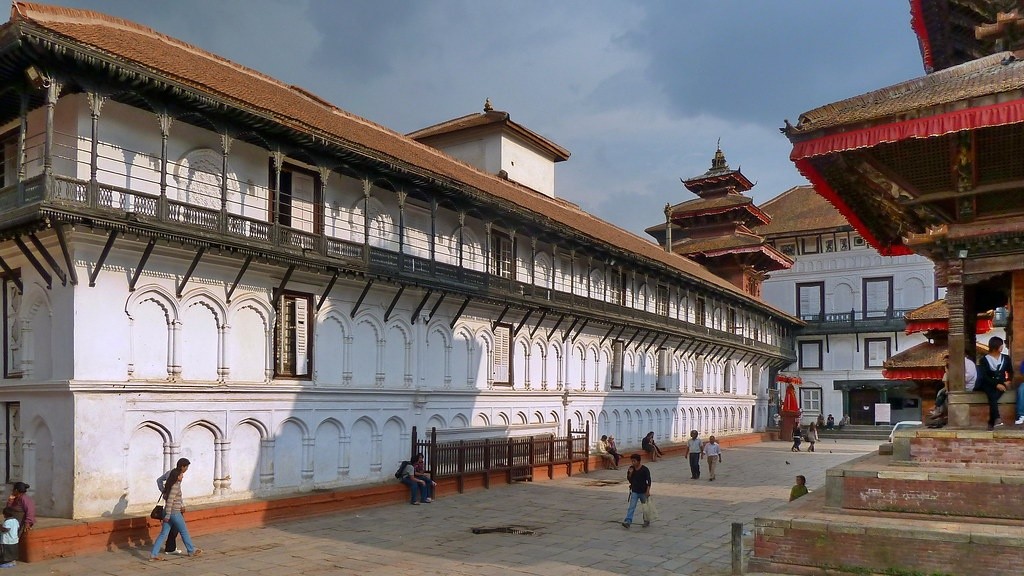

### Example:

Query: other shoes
xmin=928 ymin=405 xmax=945 ymax=419
xmin=622 ymin=522 xmax=630 ymax=529
xmin=421 ymin=499 xmax=431 ymax=503
xmin=993 ymin=417 xmax=1003 ymax=428
xmin=433 ymin=479 xmax=440 ymax=486
xmin=610 ymin=466 xmax=619 ymax=470
xmin=0 ymin=560 xmax=16 ymax=568
xmin=411 ymin=501 xmax=419 ymax=505
xmin=792 ymin=447 xmax=798 ymax=452
xmin=643 ymin=520 xmax=649 ymax=527
xmin=1015 ymin=415 xmax=1024 ymax=425
xmin=165 ymin=547 xmax=182 ymax=554
xmin=148 ymin=557 xmax=168 ymax=561
xmin=188 ymin=550 xmax=201 ymax=557
xmin=985 ymin=424 xmax=995 ymax=432
xmin=427 ymin=497 xmax=434 ymax=501
xmin=710 ymin=479 xmax=712 ymax=481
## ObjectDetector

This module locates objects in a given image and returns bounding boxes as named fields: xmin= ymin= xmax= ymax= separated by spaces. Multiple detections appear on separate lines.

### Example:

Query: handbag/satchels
xmin=151 ymin=506 xmax=165 ymax=520
xmin=804 ymin=434 xmax=810 ymax=442
xmin=643 ymin=496 xmax=658 ymax=521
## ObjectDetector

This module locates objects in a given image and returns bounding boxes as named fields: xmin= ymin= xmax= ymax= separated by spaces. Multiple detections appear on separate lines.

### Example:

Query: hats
xmin=609 ymin=435 xmax=614 ymax=439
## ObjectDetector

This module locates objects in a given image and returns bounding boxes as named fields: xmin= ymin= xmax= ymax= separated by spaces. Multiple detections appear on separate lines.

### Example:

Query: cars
xmin=889 ymin=421 xmax=922 ymax=443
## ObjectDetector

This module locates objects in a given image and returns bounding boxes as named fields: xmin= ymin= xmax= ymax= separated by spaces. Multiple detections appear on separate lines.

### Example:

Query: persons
xmin=685 ymin=430 xmax=703 ymax=480
xmin=149 ymin=468 xmax=201 ymax=561
xmin=1015 ymin=361 xmax=1024 ymax=425
xmin=979 ymin=337 xmax=1013 ymax=432
xmin=606 ymin=435 xmax=623 ymax=467
xmin=622 ymin=454 xmax=651 ymax=528
xmin=401 ymin=456 xmax=430 ymax=504
xmin=157 ymin=458 xmax=190 ymax=554
xmin=6 ymin=482 xmax=35 ymax=537
xmin=789 ymin=476 xmax=808 ymax=501
xmin=0 ymin=507 xmax=20 ymax=568
xmin=928 ymin=353 xmax=977 ymax=418
xmin=650 ymin=431 xmax=665 ymax=456
xmin=839 ymin=413 xmax=849 ymax=428
xmin=807 ymin=422 xmax=818 ymax=452
xmin=643 ymin=433 xmax=662 ymax=462
xmin=597 ymin=435 xmax=619 ymax=470
xmin=703 ymin=436 xmax=721 ymax=481
xmin=791 ymin=422 xmax=804 ymax=452
xmin=415 ymin=453 xmax=437 ymax=501
xmin=827 ymin=414 xmax=834 ymax=431
xmin=817 ymin=414 xmax=825 ymax=431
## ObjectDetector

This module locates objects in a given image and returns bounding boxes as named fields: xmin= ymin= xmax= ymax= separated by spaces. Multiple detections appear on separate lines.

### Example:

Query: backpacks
xmin=395 ymin=461 xmax=414 ymax=479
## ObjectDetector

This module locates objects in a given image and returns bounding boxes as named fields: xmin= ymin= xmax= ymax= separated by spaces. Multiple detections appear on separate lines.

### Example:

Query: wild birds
xmin=786 ymin=461 xmax=790 ymax=465
xmin=834 ymin=440 xmax=837 ymax=443
xmin=830 ymin=450 xmax=832 ymax=453
